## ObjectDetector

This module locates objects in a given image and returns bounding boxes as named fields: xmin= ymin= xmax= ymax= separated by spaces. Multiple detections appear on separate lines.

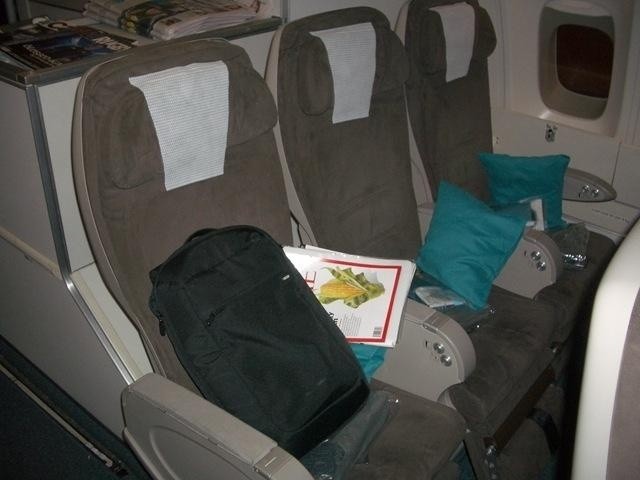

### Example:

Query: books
xmin=282 ymin=245 xmax=416 ymax=348
xmin=0 ymin=15 xmax=138 ymax=71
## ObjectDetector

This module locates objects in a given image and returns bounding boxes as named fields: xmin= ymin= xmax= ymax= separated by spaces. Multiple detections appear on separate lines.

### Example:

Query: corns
xmin=320 ymin=283 xmax=366 ymax=300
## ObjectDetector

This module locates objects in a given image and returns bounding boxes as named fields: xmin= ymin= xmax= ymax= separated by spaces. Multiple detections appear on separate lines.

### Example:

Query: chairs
xmin=69 ymin=0 xmax=640 ymax=480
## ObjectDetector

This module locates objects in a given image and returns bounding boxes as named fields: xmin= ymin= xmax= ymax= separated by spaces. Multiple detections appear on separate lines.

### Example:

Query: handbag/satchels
xmin=149 ymin=223 xmax=369 ymax=459
